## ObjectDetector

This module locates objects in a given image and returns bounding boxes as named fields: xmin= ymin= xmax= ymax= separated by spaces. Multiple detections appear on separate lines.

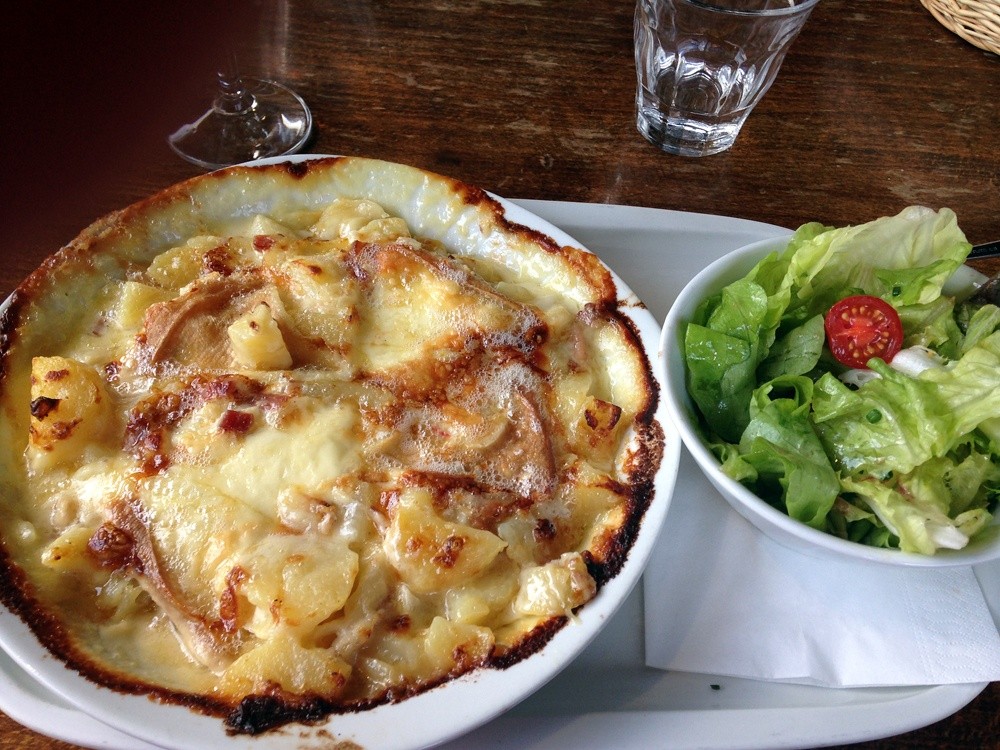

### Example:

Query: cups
xmin=633 ymin=1 xmax=818 ymax=158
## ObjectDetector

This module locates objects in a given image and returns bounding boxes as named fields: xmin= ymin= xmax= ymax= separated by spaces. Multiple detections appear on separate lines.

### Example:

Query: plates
xmin=0 ymin=155 xmax=683 ymax=750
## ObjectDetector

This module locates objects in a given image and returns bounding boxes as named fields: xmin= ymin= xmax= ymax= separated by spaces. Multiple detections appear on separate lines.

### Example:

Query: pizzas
xmin=1 ymin=154 xmax=660 ymax=728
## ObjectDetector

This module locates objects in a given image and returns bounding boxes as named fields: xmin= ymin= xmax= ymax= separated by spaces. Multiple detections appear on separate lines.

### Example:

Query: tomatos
xmin=825 ymin=294 xmax=903 ymax=369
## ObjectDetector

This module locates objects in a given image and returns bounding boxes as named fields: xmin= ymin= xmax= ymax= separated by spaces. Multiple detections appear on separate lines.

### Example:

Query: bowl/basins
xmin=658 ymin=236 xmax=998 ymax=567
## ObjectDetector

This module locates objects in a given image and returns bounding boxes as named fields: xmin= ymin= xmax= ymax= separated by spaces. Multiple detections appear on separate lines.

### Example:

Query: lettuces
xmin=681 ymin=207 xmax=1000 ymax=558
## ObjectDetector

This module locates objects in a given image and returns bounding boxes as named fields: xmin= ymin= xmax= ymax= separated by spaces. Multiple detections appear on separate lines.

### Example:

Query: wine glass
xmin=161 ymin=1 xmax=313 ymax=169
